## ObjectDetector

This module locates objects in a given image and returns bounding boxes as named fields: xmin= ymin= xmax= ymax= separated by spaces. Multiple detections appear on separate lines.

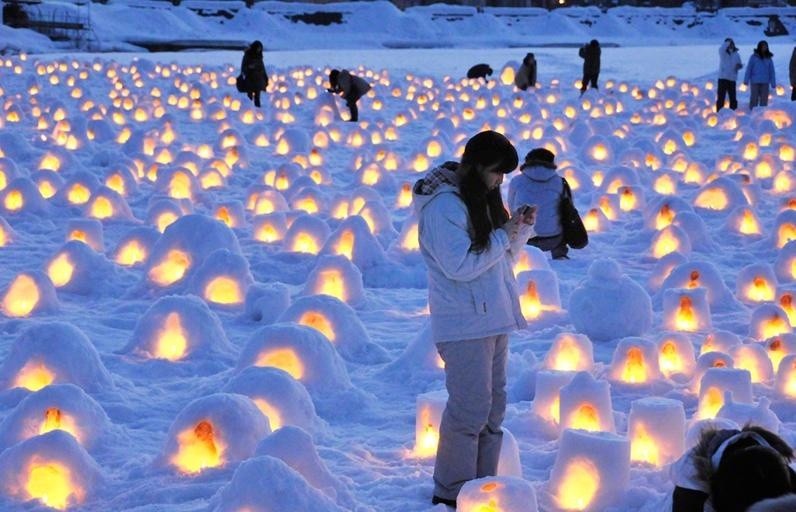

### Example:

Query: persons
xmin=716 ymin=38 xmax=796 ymax=112
xmin=515 ymin=53 xmax=537 ymax=90
xmin=241 ymin=40 xmax=269 ymax=108
xmin=579 ymin=39 xmax=601 ymax=90
xmin=410 ymin=131 xmax=539 ymax=508
xmin=327 ymin=69 xmax=372 ymax=122
xmin=467 ymin=63 xmax=493 ymax=83
xmin=507 ymin=148 xmax=572 ymax=259
xmin=670 ymin=422 xmax=796 ymax=512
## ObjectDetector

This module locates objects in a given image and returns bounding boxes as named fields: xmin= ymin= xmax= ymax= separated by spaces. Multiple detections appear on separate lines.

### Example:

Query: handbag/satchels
xmin=558 ymin=197 xmax=589 ymax=250
xmin=235 ymin=73 xmax=247 ymax=93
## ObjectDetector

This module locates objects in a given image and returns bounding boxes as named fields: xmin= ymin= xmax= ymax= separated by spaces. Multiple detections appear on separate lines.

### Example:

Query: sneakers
xmin=431 ymin=495 xmax=457 ymax=509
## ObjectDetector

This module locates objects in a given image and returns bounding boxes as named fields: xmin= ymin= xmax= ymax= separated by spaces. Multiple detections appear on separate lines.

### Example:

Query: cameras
xmin=521 ymin=204 xmax=531 ymax=216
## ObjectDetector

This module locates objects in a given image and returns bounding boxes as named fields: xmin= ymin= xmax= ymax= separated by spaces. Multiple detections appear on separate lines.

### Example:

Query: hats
xmin=519 ymin=147 xmax=557 ymax=172
